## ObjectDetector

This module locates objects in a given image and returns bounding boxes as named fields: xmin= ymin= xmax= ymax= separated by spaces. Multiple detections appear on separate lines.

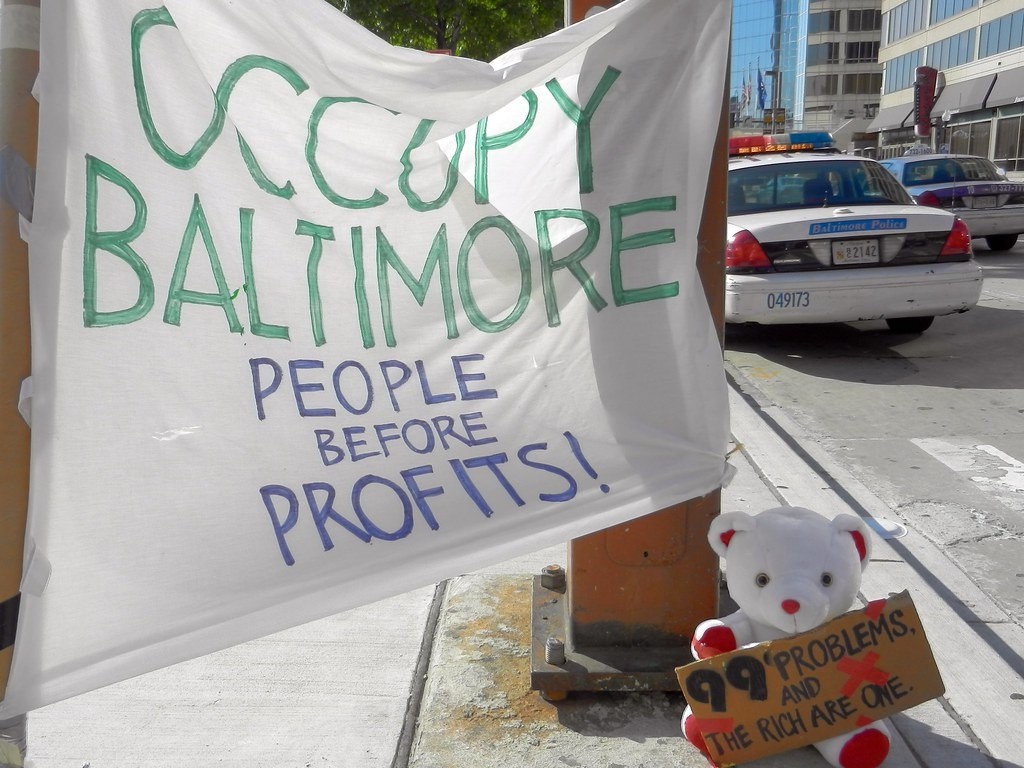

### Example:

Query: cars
xmin=878 ymin=154 xmax=1024 ymax=250
xmin=726 ymin=129 xmax=982 ymax=333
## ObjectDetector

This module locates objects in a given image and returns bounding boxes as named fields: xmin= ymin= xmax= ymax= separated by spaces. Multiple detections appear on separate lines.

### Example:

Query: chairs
xmin=728 ymin=186 xmax=745 ymax=212
xmin=932 ymin=169 xmax=950 ymax=179
xmin=803 ymin=178 xmax=833 ymax=205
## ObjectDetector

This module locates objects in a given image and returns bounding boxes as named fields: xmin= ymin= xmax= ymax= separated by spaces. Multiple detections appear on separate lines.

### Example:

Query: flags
xmin=756 ymin=69 xmax=768 ymax=110
xmin=741 ymin=75 xmax=752 ymax=110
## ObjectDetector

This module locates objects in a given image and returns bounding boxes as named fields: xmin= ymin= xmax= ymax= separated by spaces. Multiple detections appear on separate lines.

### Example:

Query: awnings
xmin=986 ymin=66 xmax=1024 ymax=109
xmin=865 ymin=102 xmax=936 ymax=134
xmin=930 ymin=73 xmax=997 ymax=118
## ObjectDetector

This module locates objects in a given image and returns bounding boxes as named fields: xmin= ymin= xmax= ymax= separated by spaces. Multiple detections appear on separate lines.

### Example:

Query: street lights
xmin=942 ymin=110 xmax=950 ymax=143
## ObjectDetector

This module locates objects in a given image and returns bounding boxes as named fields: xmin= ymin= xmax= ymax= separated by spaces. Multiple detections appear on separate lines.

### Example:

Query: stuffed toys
xmin=681 ymin=506 xmax=891 ymax=768
